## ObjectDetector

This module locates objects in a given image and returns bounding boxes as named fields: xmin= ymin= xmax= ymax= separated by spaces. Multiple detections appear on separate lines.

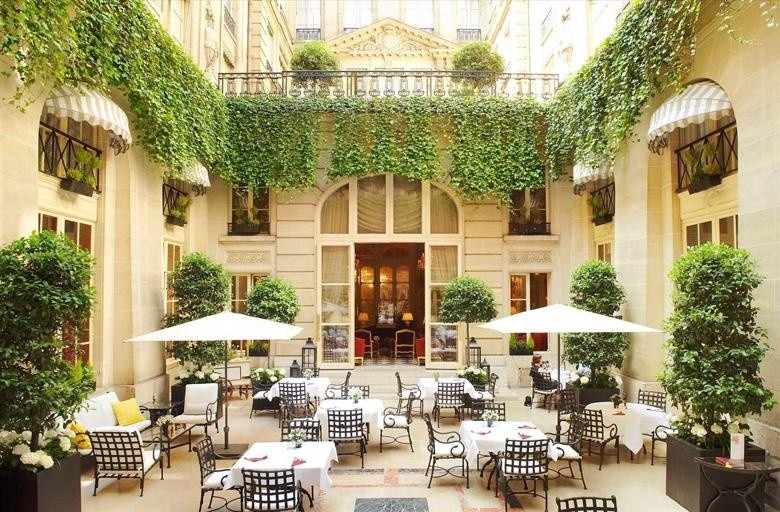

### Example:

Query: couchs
xmin=52 ymin=392 xmax=152 ymax=464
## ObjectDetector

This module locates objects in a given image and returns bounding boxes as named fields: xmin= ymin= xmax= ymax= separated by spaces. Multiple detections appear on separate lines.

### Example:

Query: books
xmin=714 ymin=455 xmax=743 ymax=469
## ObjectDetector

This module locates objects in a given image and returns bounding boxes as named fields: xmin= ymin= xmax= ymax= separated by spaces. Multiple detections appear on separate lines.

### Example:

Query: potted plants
xmin=586 ymin=196 xmax=613 ymax=226
xmin=234 ymin=207 xmax=260 ymax=233
xmin=245 ymin=277 xmax=301 ymax=398
xmin=682 ymin=143 xmax=723 ymax=195
xmin=60 ymin=149 xmax=103 ymax=198
xmin=0 ymin=230 xmax=96 ymax=512
xmin=656 ymin=242 xmax=780 ymax=512
xmin=561 ymin=260 xmax=630 ymax=414
xmin=163 ymin=251 xmax=223 ymax=426
xmin=166 ymin=195 xmax=193 ymax=227
xmin=520 ymin=206 xmax=543 ymax=233
xmin=438 ymin=275 xmax=500 ymax=393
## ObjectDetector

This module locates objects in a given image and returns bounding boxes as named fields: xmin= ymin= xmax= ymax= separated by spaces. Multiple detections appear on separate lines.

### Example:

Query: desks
xmin=692 ymin=456 xmax=780 ymax=512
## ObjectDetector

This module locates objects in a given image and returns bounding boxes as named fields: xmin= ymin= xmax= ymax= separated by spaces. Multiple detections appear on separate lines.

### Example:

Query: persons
xmin=530 ymin=351 xmax=561 ymax=408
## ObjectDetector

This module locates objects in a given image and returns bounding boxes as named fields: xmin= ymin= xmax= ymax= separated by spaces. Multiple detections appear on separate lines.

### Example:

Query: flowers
xmin=0 ymin=428 xmax=76 ymax=473
xmin=670 ymin=414 xmax=754 ymax=450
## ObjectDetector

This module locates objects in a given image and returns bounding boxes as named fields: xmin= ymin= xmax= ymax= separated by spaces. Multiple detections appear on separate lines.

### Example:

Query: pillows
xmin=64 ymin=421 xmax=92 ymax=448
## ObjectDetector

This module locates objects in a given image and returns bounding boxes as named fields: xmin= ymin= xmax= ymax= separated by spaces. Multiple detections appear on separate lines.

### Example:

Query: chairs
xmin=355 ymin=329 xmax=374 ymax=359
xmin=354 ymin=337 xmax=366 ymax=367
xmin=416 ymin=337 xmax=441 ymax=367
xmin=390 ymin=329 xmax=415 ymax=357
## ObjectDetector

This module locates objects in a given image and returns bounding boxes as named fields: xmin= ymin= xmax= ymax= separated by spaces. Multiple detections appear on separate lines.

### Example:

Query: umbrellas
xmin=476 ymin=303 xmax=665 ymax=444
xmin=123 ymin=306 xmax=305 ymax=451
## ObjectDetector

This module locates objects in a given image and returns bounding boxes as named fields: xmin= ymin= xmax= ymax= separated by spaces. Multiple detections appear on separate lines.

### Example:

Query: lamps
xmin=358 ymin=312 xmax=370 ymax=328
xmin=402 ymin=313 xmax=414 ymax=326
